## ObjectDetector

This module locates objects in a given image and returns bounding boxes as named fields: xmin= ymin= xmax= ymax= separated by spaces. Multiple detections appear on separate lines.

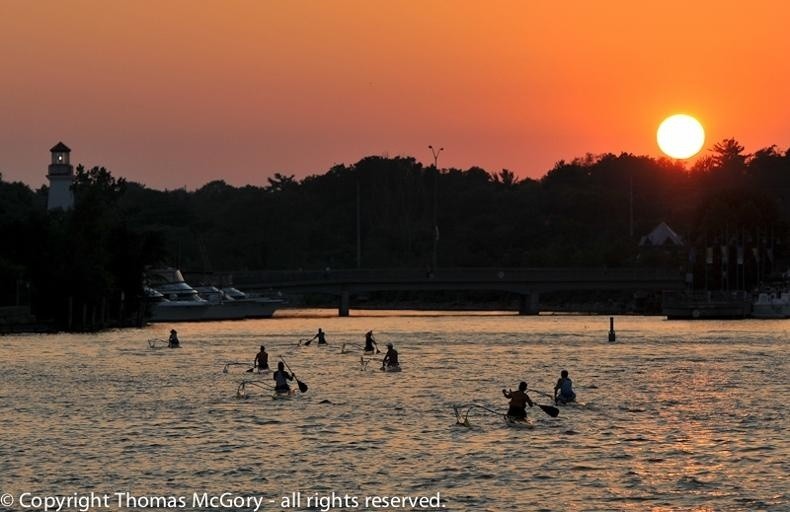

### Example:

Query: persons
xmin=363 ymin=329 xmax=378 ymax=352
xmin=382 ymin=342 xmax=402 ymax=371
xmin=273 ymin=361 xmax=295 ymax=393
xmin=502 ymin=382 xmax=532 ymax=421
xmin=168 ymin=329 xmax=180 ymax=347
xmin=553 ymin=369 xmax=576 ymax=405
xmin=254 ymin=345 xmax=269 ymax=370
xmin=305 ymin=328 xmax=328 ymax=343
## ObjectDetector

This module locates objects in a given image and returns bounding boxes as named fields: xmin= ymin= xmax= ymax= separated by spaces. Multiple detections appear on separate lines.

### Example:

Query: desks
xmin=428 ymin=143 xmax=447 ymax=272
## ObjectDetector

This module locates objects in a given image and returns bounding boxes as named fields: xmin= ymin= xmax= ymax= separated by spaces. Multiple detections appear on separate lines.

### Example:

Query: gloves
xmin=236 ymin=379 xmax=297 ymax=400
xmin=379 ymin=362 xmax=399 ymax=373
xmin=548 ymin=394 xmax=586 ymax=408
xmin=449 ymin=405 xmax=533 ymax=430
xmin=659 ymin=299 xmax=757 ymax=321
xmin=338 ymin=340 xmax=378 ymax=356
xmin=133 ymin=256 xmax=289 ymax=321
xmin=221 ymin=360 xmax=273 ymax=375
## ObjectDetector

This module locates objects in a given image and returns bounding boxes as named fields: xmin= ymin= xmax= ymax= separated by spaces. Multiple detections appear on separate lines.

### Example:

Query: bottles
xmin=385 ymin=342 xmax=394 ymax=347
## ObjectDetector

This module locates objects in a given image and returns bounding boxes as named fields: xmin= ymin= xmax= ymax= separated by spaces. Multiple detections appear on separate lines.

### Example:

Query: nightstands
xmin=279 ymin=355 xmax=308 ymax=393
xmin=372 ymin=333 xmax=380 ymax=354
xmin=506 ymin=391 xmax=559 ymax=418
xmin=247 ymin=365 xmax=259 ymax=373
xmin=305 ymin=339 xmax=314 ymax=345
xmin=380 ymin=366 xmax=385 ymax=371
